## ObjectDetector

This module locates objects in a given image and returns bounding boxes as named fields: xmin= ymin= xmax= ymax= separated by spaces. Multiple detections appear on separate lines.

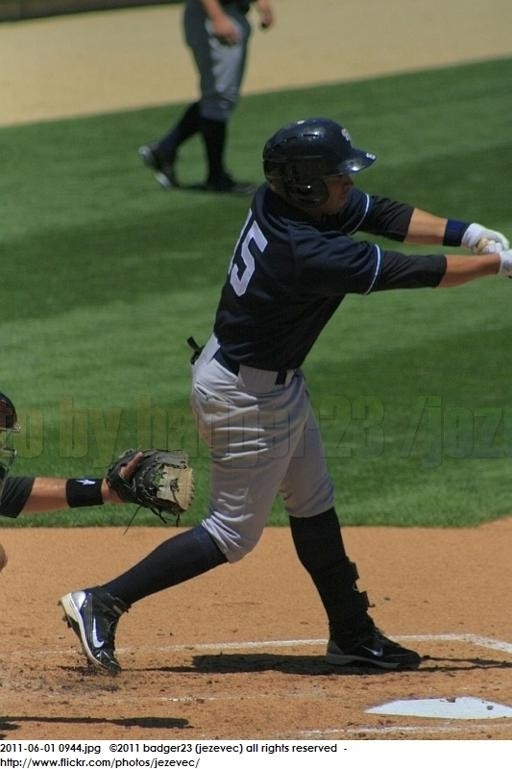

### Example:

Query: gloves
xmin=495 ymin=250 xmax=511 ymax=277
xmin=461 ymin=219 xmax=510 ymax=251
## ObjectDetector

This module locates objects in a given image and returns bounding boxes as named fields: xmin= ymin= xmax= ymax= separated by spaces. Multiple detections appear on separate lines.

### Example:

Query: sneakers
xmin=57 ymin=588 xmax=127 ymax=675
xmin=138 ymin=145 xmax=179 ymax=191
xmin=325 ymin=626 xmax=421 ymax=671
xmin=204 ymin=179 xmax=254 ymax=196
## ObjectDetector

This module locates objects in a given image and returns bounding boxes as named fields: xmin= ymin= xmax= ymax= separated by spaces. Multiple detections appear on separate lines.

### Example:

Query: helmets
xmin=262 ymin=117 xmax=378 ymax=201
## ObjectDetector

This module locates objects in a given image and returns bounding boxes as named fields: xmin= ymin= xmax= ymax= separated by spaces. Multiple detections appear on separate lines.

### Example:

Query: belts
xmin=205 ymin=335 xmax=295 ymax=386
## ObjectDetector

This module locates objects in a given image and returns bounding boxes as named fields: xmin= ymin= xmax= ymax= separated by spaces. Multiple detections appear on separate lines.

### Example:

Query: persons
xmin=138 ymin=0 xmax=273 ymax=195
xmin=0 ymin=391 xmax=197 ymax=572
xmin=57 ymin=118 xmax=512 ymax=677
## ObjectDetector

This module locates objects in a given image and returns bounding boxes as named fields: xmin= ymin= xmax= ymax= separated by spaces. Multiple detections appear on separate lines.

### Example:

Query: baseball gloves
xmin=105 ymin=449 xmax=196 ymax=515
